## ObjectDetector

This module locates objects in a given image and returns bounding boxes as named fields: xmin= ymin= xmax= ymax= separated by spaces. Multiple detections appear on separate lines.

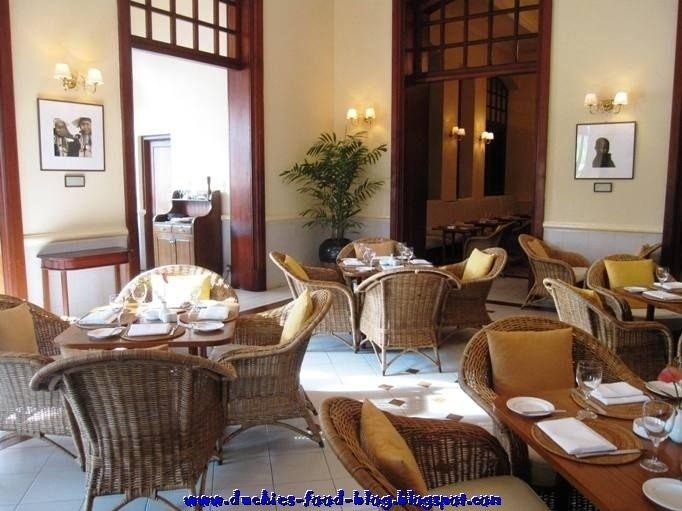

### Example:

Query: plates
xmin=192 ymin=299 xmax=216 ymax=307
xmin=645 ymin=381 xmax=682 ymax=399
xmin=642 ymin=476 xmax=682 ymax=511
xmin=356 ymin=267 xmax=374 ymax=272
xmin=87 ymin=327 xmax=122 ymax=338
xmin=109 ymin=294 xmax=125 ymax=329
xmin=397 ymin=256 xmax=406 ymax=259
xmin=132 ymin=283 xmax=147 ymax=317
xmin=506 ymin=396 xmax=555 ymax=417
xmin=623 ymin=286 xmax=647 ymax=293
xmin=193 ymin=320 xmax=224 ymax=332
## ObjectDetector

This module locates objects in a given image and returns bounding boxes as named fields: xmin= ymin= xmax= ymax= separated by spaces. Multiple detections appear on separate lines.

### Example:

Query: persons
xmin=54 ymin=119 xmax=67 ymax=156
xmin=592 ymin=136 xmax=616 ymax=168
xmin=79 ymin=117 xmax=91 ymax=136
xmin=69 ymin=133 xmax=81 ymax=156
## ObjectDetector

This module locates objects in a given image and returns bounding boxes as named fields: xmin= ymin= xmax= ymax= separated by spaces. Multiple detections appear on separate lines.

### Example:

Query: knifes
xmin=574 ymin=390 xmax=608 ymax=416
xmin=576 ymin=447 xmax=651 ymax=459
xmin=169 ymin=323 xmax=179 ymax=336
xmin=644 ymin=380 xmax=675 ymax=399
xmin=522 ymin=409 xmax=566 ymax=415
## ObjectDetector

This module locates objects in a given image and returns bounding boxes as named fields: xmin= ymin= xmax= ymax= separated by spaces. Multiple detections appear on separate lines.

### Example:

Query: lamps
xmin=480 ymin=131 xmax=495 ymax=145
xmin=52 ymin=63 xmax=103 ymax=95
xmin=346 ymin=107 xmax=376 ymax=128
xmin=449 ymin=126 xmax=466 ymax=142
xmin=583 ymin=90 xmax=629 ymax=114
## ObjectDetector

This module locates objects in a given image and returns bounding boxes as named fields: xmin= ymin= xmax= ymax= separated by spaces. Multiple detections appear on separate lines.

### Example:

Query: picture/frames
xmin=574 ymin=121 xmax=637 ymax=180
xmin=37 ymin=97 xmax=106 ymax=173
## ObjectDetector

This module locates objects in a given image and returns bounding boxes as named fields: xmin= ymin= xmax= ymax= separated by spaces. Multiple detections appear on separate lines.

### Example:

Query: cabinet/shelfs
xmin=153 ymin=191 xmax=223 ymax=276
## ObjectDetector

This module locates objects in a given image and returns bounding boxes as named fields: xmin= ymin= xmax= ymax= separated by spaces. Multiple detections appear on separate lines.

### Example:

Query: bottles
xmin=388 ymin=253 xmax=394 ymax=265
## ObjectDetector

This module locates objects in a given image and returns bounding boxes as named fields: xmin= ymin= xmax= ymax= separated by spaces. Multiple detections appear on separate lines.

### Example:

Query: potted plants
xmin=278 ymin=130 xmax=388 ymax=286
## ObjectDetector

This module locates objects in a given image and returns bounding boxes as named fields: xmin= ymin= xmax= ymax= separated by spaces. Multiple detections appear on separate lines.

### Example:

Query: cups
xmin=162 ymin=312 xmax=177 ymax=323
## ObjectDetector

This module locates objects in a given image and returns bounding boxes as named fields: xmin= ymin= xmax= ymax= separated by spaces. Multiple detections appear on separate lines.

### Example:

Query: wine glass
xmin=405 ymin=247 xmax=413 ymax=265
xmin=576 ymin=360 xmax=602 ymax=421
xmin=189 ymin=287 xmax=202 ymax=308
xmin=359 ymin=243 xmax=367 ymax=261
xmin=398 ymin=243 xmax=407 ymax=263
xmin=656 ymin=267 xmax=669 ymax=290
xmin=639 ymin=399 xmax=675 ymax=473
xmin=367 ymin=248 xmax=376 ymax=271
